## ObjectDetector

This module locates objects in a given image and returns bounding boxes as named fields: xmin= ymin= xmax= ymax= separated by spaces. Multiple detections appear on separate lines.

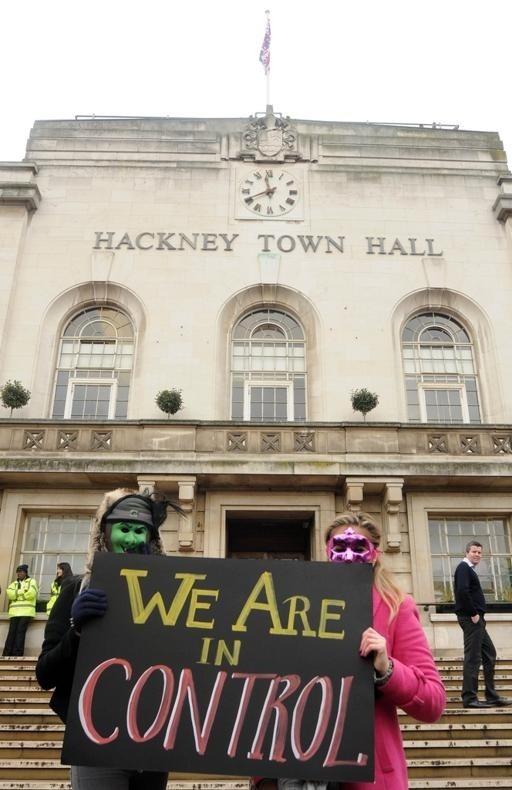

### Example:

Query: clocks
xmin=237 ymin=165 xmax=300 ymax=218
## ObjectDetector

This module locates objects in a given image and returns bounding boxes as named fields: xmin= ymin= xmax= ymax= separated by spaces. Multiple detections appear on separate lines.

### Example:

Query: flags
xmin=258 ymin=21 xmax=272 ymax=72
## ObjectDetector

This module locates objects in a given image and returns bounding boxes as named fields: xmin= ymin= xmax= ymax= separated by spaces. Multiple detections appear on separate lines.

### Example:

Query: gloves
xmin=70 ymin=588 xmax=108 ymax=631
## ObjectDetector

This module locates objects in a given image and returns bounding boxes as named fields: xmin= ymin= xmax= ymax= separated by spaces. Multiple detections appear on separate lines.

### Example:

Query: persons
xmin=33 ymin=484 xmax=171 ymax=787
xmin=45 ymin=561 xmax=74 ymax=620
xmin=1 ymin=563 xmax=39 ymax=658
xmin=246 ymin=510 xmax=449 ymax=790
xmin=451 ymin=538 xmax=511 ymax=709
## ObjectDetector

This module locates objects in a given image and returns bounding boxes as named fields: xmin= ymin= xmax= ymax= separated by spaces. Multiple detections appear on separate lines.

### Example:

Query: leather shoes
xmin=464 ymin=695 xmax=512 ymax=708
